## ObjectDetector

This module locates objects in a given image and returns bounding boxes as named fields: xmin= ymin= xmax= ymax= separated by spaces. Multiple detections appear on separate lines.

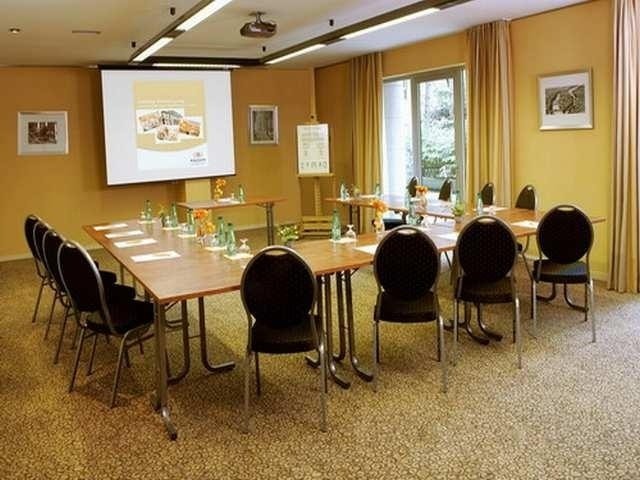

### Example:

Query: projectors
xmin=239 ymin=21 xmax=277 ymax=38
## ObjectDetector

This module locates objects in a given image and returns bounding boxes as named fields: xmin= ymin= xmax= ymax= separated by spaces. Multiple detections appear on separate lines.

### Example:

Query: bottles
xmin=338 ymin=175 xmax=484 ymax=224
xmin=144 ymin=200 xmax=236 ymax=258
xmin=237 ymin=182 xmax=245 ymax=203
xmin=331 ymin=209 xmax=342 ymax=240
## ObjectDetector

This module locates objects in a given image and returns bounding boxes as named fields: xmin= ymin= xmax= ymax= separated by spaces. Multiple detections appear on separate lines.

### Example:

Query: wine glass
xmin=237 ymin=238 xmax=251 ymax=258
xmin=345 ymin=224 xmax=357 ymax=241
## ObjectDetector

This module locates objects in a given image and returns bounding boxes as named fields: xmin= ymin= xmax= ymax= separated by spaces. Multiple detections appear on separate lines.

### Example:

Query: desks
xmin=83 ymin=213 xmax=243 ymax=387
xmin=176 ymin=195 xmax=288 ymax=252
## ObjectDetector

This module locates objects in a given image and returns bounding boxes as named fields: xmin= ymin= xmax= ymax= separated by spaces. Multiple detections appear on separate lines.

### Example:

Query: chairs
xmin=23 ymin=210 xmax=188 ymax=410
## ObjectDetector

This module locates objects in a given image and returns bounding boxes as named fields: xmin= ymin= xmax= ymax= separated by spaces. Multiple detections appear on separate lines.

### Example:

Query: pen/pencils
xmin=128 ymin=241 xmax=140 ymax=243
xmin=155 ymin=253 xmax=170 ymax=256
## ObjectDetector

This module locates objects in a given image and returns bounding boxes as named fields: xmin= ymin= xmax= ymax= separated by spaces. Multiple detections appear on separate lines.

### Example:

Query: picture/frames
xmin=16 ymin=110 xmax=70 ymax=157
xmin=249 ymin=104 xmax=279 ymax=145
xmin=534 ymin=65 xmax=596 ymax=132
